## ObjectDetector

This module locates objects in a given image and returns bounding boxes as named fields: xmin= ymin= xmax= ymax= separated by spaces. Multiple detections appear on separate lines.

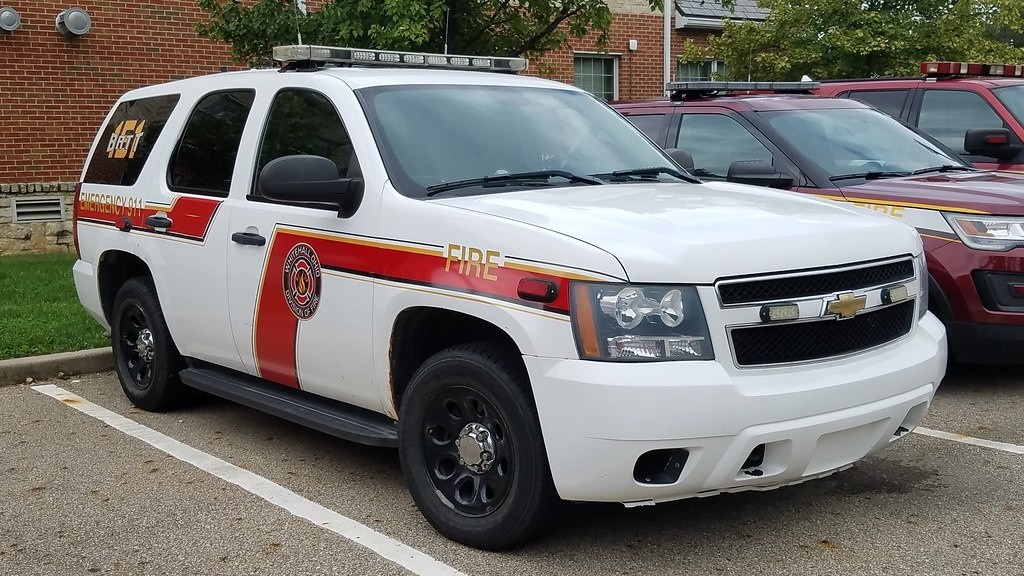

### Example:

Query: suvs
xmin=811 ymin=61 xmax=1024 ymax=179
xmin=598 ymin=83 xmax=1024 ymax=376
xmin=72 ymin=42 xmax=949 ymax=554
